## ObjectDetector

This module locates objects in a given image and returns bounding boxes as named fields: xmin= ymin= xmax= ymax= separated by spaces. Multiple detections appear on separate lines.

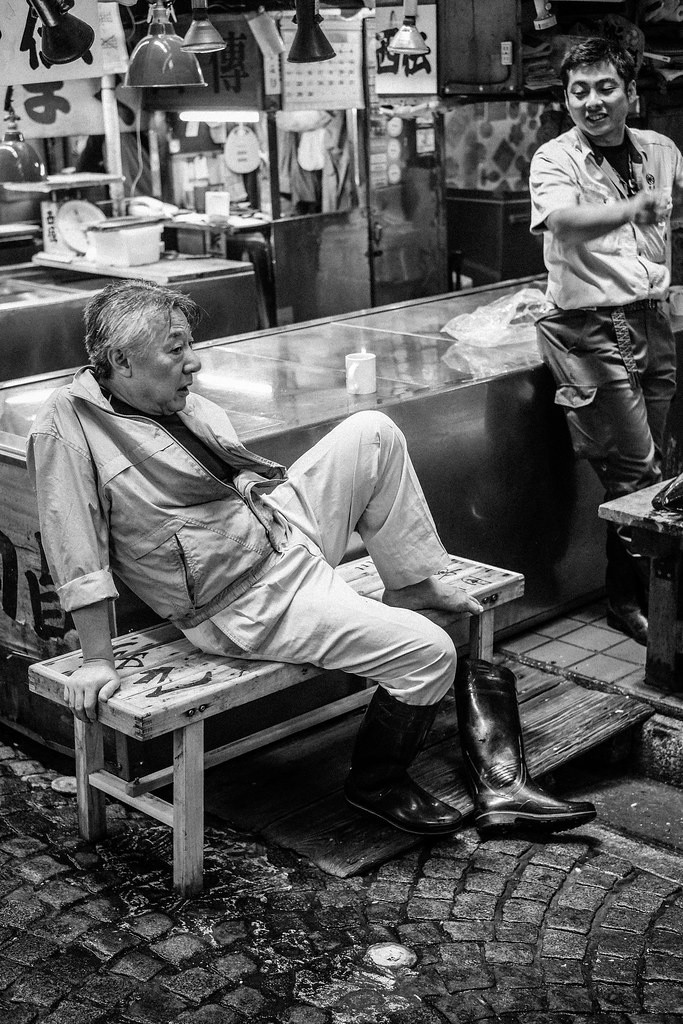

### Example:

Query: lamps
xmin=534 ymin=0 xmax=558 ymax=29
xmin=287 ymin=0 xmax=337 ymax=64
xmin=244 ymin=5 xmax=286 ymax=59
xmin=387 ymin=0 xmax=431 ymax=55
xmin=125 ymin=0 xmax=209 ymax=88
xmin=0 ymin=104 xmax=48 ymax=184
xmin=180 ymin=0 xmax=227 ymax=54
xmin=29 ymin=0 xmax=96 ymax=63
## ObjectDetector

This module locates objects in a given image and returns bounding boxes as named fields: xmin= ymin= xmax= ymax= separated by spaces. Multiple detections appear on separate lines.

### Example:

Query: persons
xmin=26 ymin=277 xmax=491 ymax=837
xmin=527 ymin=35 xmax=683 ymax=647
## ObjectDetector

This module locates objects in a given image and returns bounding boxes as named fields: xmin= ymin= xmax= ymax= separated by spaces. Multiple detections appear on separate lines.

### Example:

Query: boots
xmin=455 ymin=654 xmax=597 ymax=835
xmin=604 ymin=520 xmax=652 ymax=647
xmin=339 ymin=684 xmax=466 ymax=836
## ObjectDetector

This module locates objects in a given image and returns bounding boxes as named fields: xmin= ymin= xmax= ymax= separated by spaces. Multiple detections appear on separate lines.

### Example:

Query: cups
xmin=205 ymin=191 xmax=229 ymax=217
xmin=345 ymin=353 xmax=376 ymax=395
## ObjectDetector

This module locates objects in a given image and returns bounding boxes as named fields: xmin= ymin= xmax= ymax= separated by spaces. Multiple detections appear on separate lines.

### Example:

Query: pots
xmin=85 ymin=215 xmax=165 ymax=268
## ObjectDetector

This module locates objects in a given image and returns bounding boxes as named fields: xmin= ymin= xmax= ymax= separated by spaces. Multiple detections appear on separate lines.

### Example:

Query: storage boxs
xmin=84 ymin=215 xmax=165 ymax=268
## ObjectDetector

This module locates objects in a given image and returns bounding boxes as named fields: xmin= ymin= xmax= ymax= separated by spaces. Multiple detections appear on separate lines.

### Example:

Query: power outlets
xmin=500 ymin=41 xmax=513 ymax=66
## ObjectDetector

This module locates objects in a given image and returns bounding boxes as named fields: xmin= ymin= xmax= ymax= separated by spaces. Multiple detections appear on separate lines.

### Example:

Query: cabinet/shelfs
xmin=435 ymin=0 xmax=563 ymax=102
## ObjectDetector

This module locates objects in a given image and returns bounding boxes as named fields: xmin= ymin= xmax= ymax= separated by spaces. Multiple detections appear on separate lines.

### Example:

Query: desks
xmin=165 ymin=210 xmax=270 ymax=259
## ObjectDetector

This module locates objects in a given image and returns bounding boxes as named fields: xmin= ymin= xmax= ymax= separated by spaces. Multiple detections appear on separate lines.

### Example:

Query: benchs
xmin=598 ymin=478 xmax=683 ymax=693
xmin=28 ymin=553 xmax=525 ymax=898
